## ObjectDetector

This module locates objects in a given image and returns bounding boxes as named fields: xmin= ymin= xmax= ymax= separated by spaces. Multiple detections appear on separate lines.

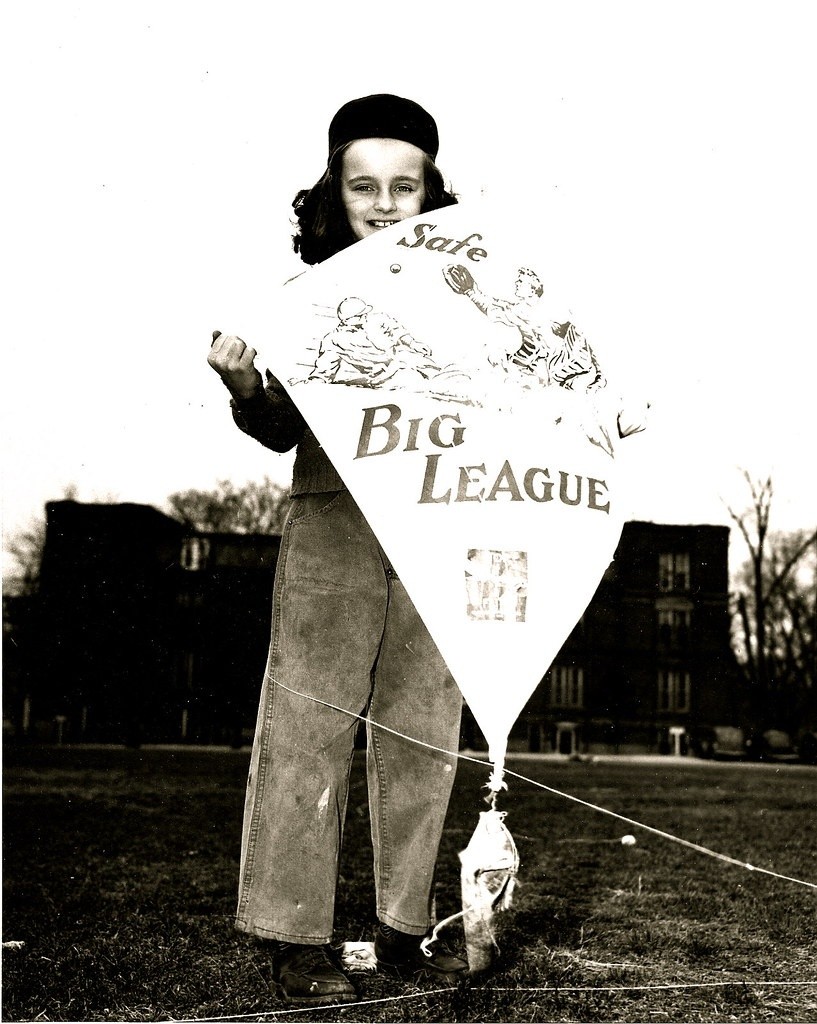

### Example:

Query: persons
xmin=209 ymin=94 xmax=650 ymax=1005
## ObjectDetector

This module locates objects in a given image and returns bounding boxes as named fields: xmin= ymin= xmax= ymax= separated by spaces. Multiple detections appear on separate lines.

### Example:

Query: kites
xmin=225 ymin=190 xmax=645 ymax=932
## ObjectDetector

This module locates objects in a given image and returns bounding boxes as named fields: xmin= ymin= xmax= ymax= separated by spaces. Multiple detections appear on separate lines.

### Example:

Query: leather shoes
xmin=271 ymin=943 xmax=357 ymax=1003
xmin=374 ymin=929 xmax=470 ymax=985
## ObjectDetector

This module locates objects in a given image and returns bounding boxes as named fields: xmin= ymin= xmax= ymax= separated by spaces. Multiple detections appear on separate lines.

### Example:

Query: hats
xmin=327 ymin=92 xmax=441 ymax=171
